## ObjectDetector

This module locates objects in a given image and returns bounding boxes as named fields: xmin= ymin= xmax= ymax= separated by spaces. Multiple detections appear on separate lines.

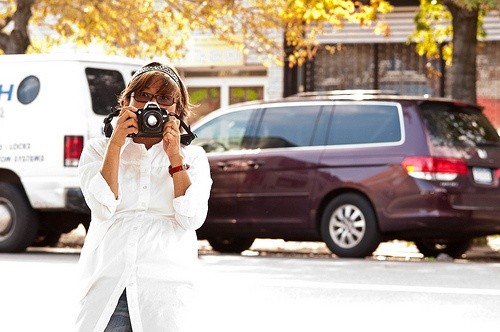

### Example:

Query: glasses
xmin=130 ymin=92 xmax=178 ymax=106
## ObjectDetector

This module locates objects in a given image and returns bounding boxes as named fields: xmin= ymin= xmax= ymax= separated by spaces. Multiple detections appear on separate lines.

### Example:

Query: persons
xmin=75 ymin=62 xmax=212 ymax=332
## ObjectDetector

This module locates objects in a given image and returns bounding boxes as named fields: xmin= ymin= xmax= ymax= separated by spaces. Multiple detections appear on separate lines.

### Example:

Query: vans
xmin=0 ymin=55 xmax=154 ymax=252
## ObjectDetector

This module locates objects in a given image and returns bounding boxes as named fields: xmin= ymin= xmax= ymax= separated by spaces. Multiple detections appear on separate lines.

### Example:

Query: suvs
xmin=181 ymin=89 xmax=500 ymax=259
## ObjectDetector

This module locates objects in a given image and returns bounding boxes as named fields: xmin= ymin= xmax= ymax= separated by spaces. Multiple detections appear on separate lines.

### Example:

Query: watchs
xmin=169 ymin=164 xmax=189 ymax=178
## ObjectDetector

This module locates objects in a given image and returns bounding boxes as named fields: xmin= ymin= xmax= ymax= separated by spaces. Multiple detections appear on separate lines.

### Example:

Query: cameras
xmin=129 ymin=102 xmax=170 ymax=138
